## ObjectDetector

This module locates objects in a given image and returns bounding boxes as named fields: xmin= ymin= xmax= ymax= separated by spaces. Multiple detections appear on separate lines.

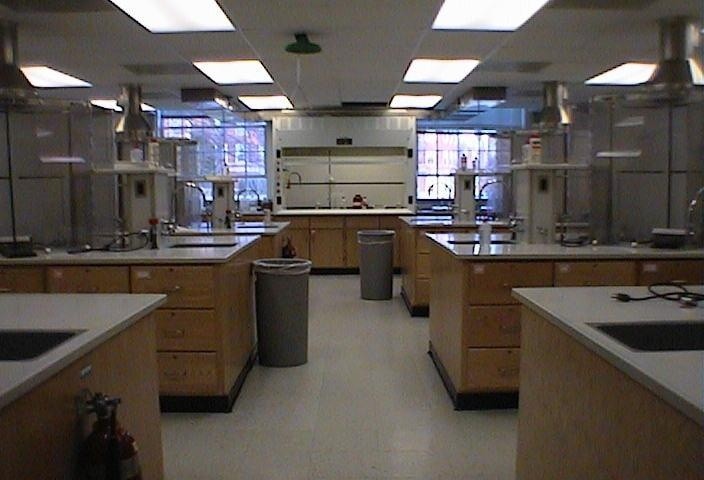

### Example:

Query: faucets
xmin=185 ymin=181 xmax=261 ymax=207
xmin=479 ymin=181 xmax=509 ymax=200
xmin=688 ymin=188 xmax=704 ymax=235
xmin=287 ymin=171 xmax=301 ymax=189
xmin=428 ymin=182 xmax=452 ymax=202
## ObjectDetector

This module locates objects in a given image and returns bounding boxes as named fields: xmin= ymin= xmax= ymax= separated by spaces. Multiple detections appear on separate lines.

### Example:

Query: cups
xmin=478 ymin=223 xmax=492 ymax=249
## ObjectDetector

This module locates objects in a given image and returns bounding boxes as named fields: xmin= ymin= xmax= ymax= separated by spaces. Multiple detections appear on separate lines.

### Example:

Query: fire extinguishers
xmin=82 ymin=390 xmax=143 ymax=479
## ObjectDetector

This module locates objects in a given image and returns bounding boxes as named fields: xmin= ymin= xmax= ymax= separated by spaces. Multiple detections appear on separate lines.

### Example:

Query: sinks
xmin=236 ymin=225 xmax=280 ymax=229
xmin=448 ymin=239 xmax=518 ymax=245
xmin=589 ymin=319 xmax=704 ymax=353
xmin=410 ymin=218 xmax=452 ymax=223
xmin=170 ymin=243 xmax=238 ymax=249
xmin=0 ymin=325 xmax=87 ymax=362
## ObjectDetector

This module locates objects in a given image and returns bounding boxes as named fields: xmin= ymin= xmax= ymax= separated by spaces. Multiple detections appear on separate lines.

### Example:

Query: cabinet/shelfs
xmin=243 ymin=215 xmax=403 ymax=273
xmin=0 ymin=241 xmax=254 ymax=412
xmin=398 ymin=219 xmax=512 ymax=315
xmin=427 ymin=241 xmax=704 ymax=411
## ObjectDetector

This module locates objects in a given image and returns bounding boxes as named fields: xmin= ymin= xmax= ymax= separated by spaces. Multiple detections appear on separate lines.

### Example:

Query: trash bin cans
xmin=252 ymin=257 xmax=312 ymax=367
xmin=356 ymin=230 xmax=395 ymax=300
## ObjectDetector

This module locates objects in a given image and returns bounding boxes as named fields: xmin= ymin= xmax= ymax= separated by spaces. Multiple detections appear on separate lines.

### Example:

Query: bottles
xmin=131 ymin=138 xmax=160 ymax=164
xmin=521 ymin=133 xmax=541 ymax=162
xmin=460 ymin=154 xmax=468 ymax=170
xmin=340 ymin=194 xmax=368 ymax=209
xmin=263 ymin=206 xmax=272 ymax=225
